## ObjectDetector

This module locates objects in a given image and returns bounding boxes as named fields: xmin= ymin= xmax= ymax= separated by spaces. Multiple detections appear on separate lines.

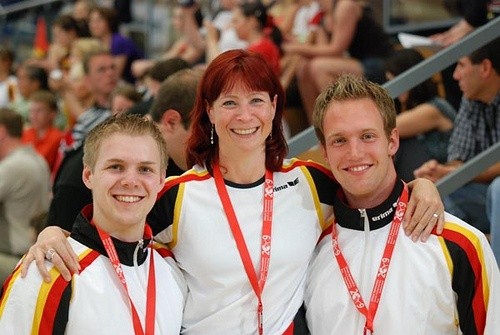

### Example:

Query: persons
xmin=0 ymin=0 xmax=500 ymax=287
xmin=282 ymin=72 xmax=500 ymax=335
xmin=20 ymin=48 xmax=445 ymax=335
xmin=0 ymin=112 xmax=188 ymax=335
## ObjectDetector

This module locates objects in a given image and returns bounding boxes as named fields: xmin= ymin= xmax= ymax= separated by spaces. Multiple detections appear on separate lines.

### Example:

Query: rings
xmin=46 ymin=249 xmax=56 ymax=262
xmin=432 ymin=213 xmax=439 ymax=219
